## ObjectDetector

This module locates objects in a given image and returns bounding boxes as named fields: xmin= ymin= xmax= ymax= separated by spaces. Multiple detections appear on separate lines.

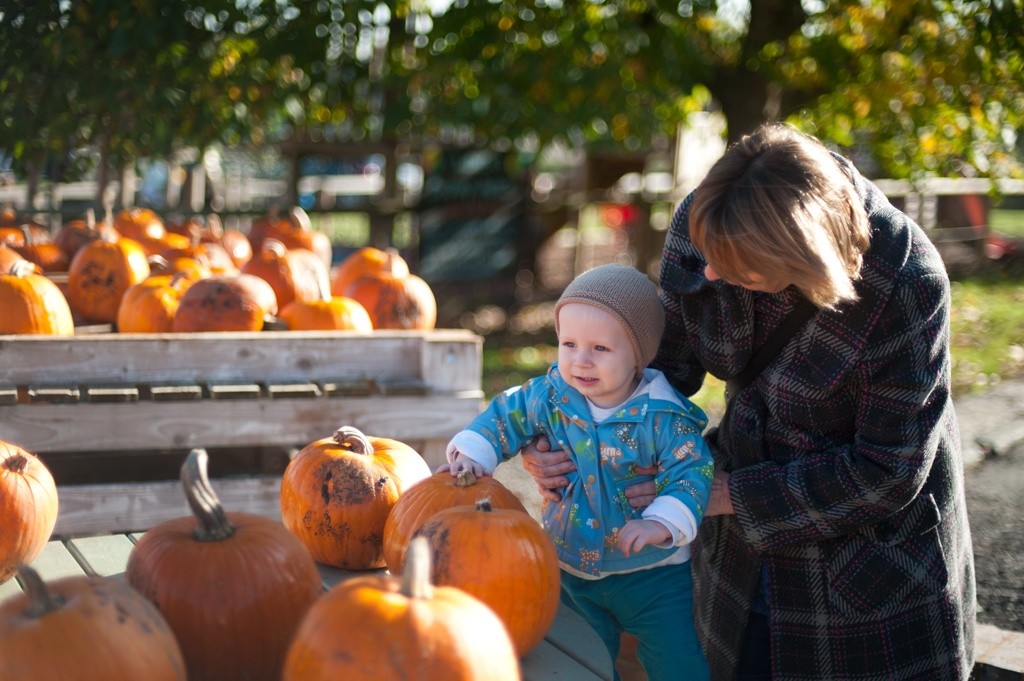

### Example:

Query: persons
xmin=447 ymin=263 xmax=717 ymax=681
xmin=518 ymin=126 xmax=977 ymax=681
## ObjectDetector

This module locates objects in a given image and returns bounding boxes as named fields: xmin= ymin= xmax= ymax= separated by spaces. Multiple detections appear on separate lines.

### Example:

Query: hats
xmin=554 ymin=263 xmax=666 ymax=375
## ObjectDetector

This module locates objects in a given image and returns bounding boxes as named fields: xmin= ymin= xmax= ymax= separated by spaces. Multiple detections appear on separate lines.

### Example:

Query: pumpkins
xmin=1 ymin=423 xmax=560 ymax=680
xmin=0 ymin=201 xmax=437 ymax=337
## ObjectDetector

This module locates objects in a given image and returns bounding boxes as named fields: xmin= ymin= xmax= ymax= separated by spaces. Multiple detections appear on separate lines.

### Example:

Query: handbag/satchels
xmin=705 ymin=427 xmax=719 ymax=448
